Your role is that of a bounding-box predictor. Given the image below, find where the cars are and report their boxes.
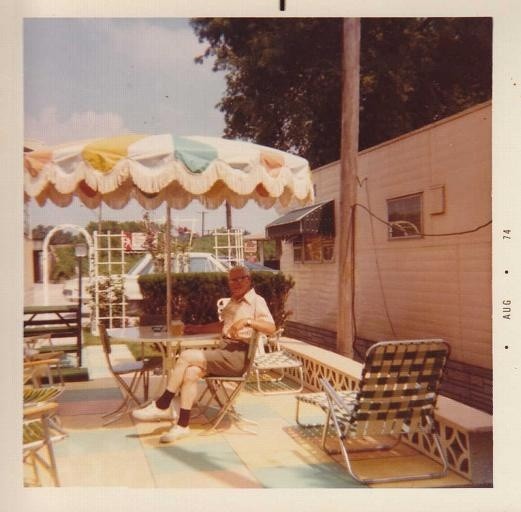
[61,252,280,307]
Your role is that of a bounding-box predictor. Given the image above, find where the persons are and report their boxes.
[130,264,278,443]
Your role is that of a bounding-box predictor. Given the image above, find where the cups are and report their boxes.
[171,318,185,337]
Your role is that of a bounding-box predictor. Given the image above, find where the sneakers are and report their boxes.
[132,400,176,422]
[158,423,193,443]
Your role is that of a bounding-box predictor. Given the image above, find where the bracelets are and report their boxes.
[248,319,252,326]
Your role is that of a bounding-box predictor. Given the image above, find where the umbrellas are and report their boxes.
[24,129,317,387]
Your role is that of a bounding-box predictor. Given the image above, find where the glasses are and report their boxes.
[227,276,248,285]
